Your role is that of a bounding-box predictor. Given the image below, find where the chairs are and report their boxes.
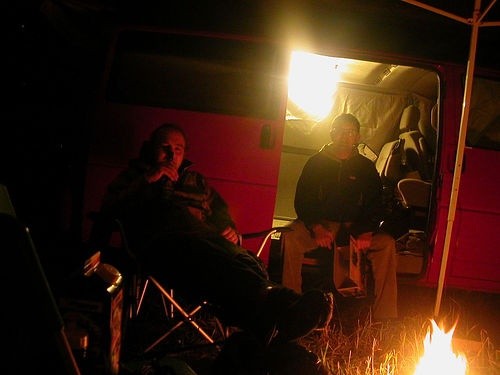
[98,182,243,366]
[373,104,434,226]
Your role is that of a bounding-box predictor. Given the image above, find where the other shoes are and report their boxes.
[272,285,336,346]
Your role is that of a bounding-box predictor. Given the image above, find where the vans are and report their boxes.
[72,26,500,306]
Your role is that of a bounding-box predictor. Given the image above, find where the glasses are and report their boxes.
[334,128,357,142]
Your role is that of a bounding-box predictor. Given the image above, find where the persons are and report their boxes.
[275,114,401,325]
[72,123,337,351]
